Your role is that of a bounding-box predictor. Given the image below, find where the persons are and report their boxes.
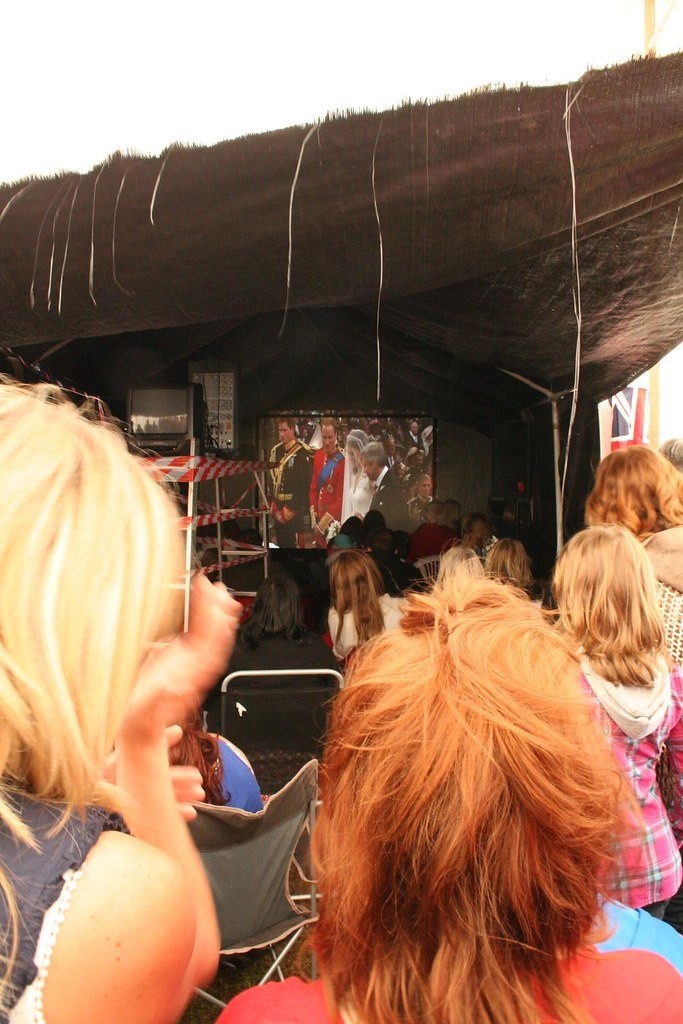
[327,550,410,663]
[355,441,407,531]
[403,474,433,527]
[583,440,683,670]
[486,481,555,595]
[294,417,433,500]
[216,577,683,1024]
[225,574,342,676]
[341,429,374,525]
[309,417,346,549]
[544,522,683,921]
[225,498,534,638]
[165,692,264,813]
[266,418,318,548]
[0,378,222,1024]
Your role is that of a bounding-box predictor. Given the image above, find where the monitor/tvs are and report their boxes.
[128,383,203,440]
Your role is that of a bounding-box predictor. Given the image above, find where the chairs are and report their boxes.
[179,755,321,1010]
[219,669,345,787]
